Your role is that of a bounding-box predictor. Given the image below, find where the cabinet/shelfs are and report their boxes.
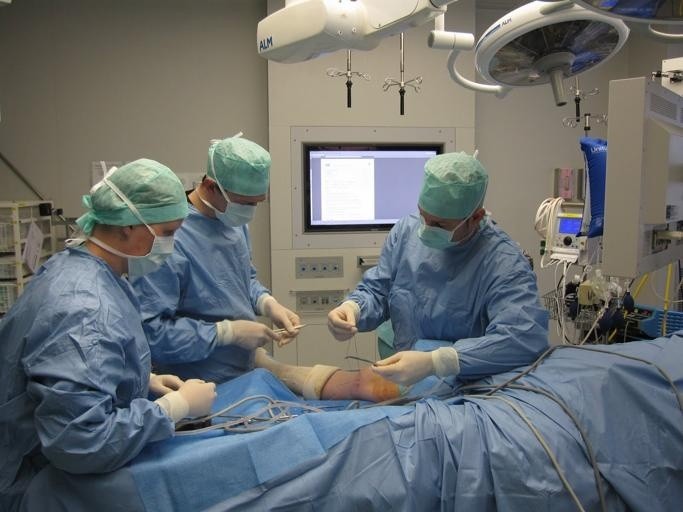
[1,196,56,322]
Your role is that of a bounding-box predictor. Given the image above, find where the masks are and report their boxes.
[416,223,460,250]
[125,235,174,278]
[213,202,257,228]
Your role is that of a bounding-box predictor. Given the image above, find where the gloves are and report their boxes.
[325,300,361,344]
[214,318,281,352]
[369,345,461,388]
[256,292,300,349]
[148,372,184,396]
[154,379,217,424]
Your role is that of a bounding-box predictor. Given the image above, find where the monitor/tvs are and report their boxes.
[304,146,441,231]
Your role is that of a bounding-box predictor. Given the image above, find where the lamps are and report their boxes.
[473,0,633,108]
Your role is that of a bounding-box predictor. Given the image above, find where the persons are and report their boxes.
[0,156,220,512]
[244,343,478,403]
[324,150,551,400]
[114,129,303,402]
